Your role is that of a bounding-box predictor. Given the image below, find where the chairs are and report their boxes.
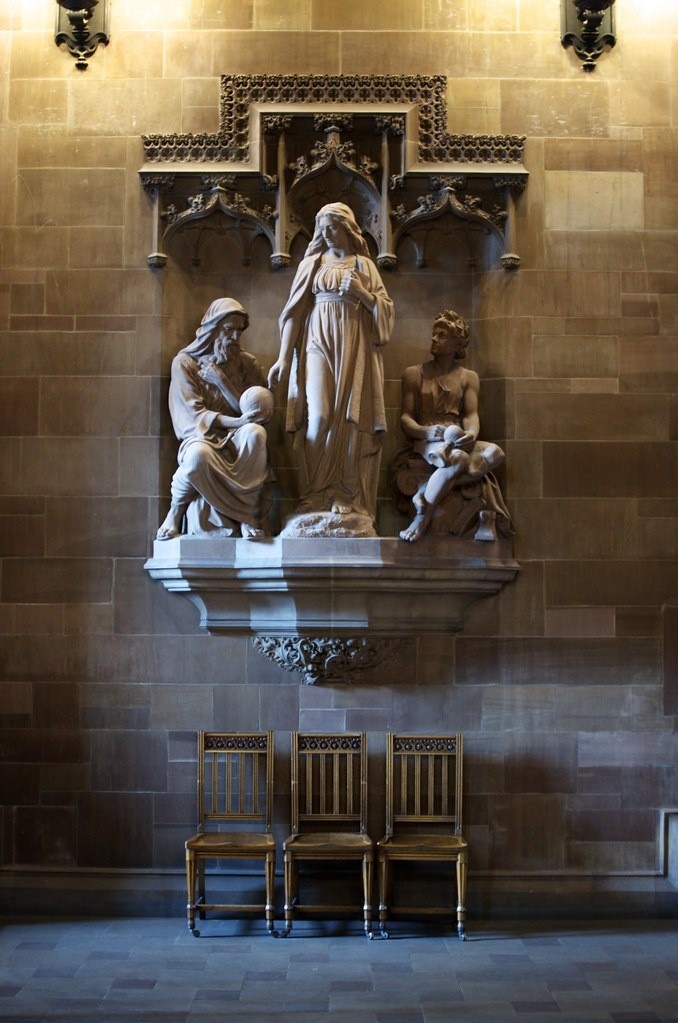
[280,732,375,938]
[376,732,468,942]
[185,730,280,939]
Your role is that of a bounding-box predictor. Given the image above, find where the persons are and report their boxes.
[396,309,507,545]
[156,295,275,542]
[266,200,394,520]
